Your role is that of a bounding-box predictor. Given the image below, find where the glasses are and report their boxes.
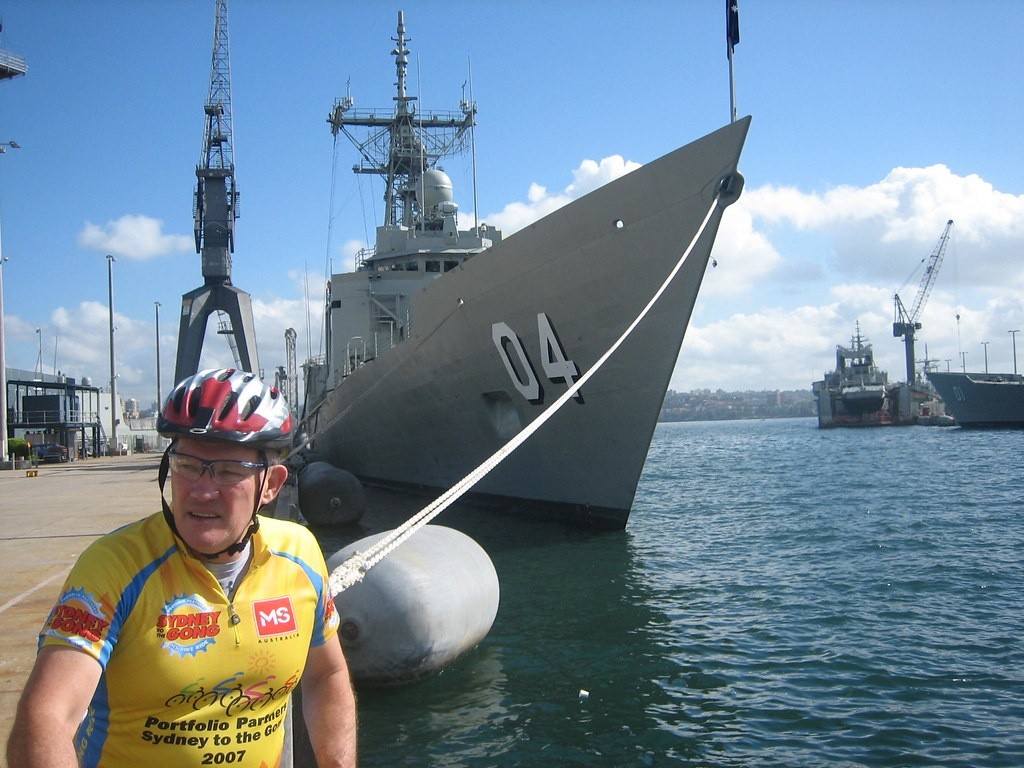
[167,446,266,485]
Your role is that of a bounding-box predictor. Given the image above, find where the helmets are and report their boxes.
[156,369,294,448]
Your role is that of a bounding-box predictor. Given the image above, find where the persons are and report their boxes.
[8,370,360,768]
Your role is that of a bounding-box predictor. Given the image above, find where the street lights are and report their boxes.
[154,302,162,416]
[981,342,990,373]
[106,254,116,453]
[1009,330,1019,374]
[960,352,968,373]
[944,359,952,372]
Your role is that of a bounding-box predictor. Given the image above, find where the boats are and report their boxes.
[926,372,1024,430]
[816,319,895,427]
[289,1,753,535]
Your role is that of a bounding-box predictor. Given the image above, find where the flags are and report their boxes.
[726,0,740,58]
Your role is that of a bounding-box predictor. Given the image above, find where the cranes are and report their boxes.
[894,219,954,387]
